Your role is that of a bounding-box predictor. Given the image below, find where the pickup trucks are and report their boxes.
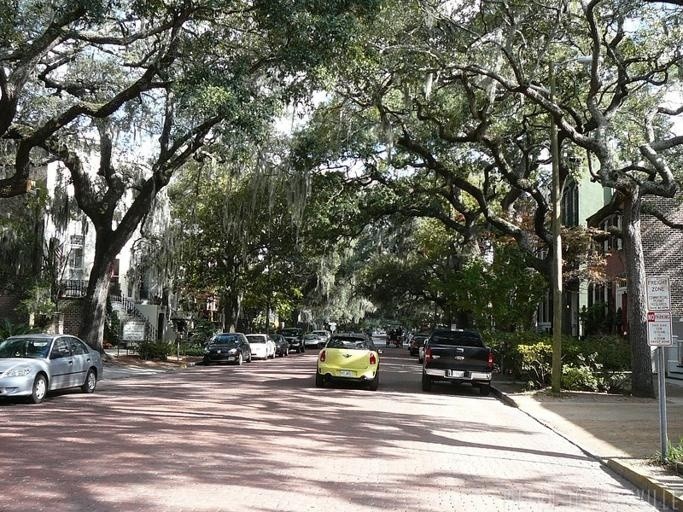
[421,331,496,396]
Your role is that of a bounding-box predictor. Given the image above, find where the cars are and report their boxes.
[408,335,429,363]
[0,334,104,404]
[204,329,332,365]
[315,333,383,391]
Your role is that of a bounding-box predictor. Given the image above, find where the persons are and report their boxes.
[386,325,404,348]
